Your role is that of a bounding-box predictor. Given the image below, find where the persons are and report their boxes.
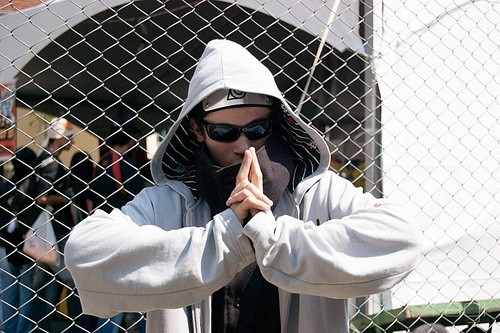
[64,39,423,332]
[0,115,152,331]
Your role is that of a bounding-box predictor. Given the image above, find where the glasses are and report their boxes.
[199,118,275,143]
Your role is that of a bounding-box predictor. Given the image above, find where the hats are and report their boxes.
[192,88,279,117]
[42,116,74,149]
